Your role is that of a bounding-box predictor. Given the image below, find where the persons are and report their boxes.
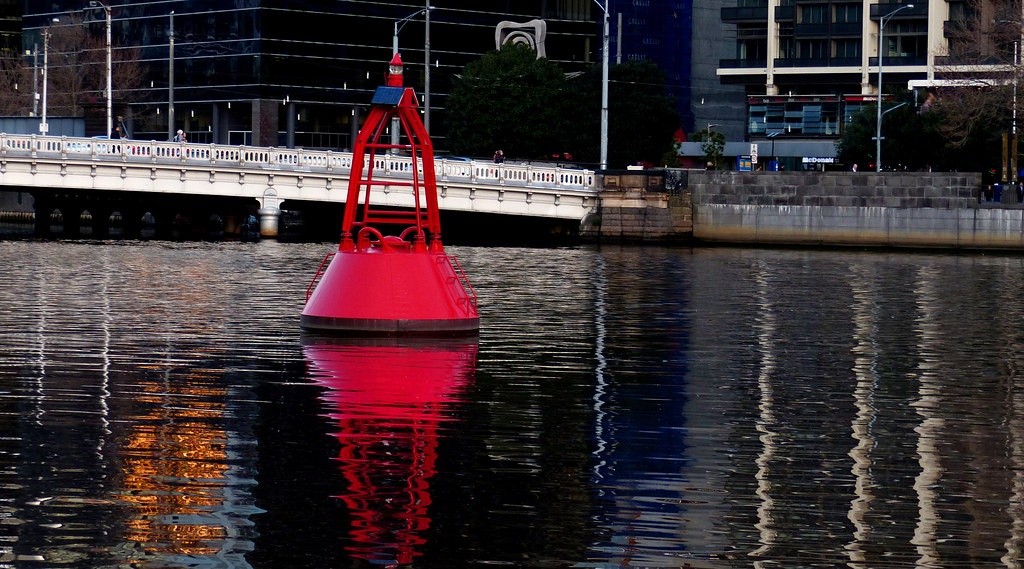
[491,149,504,177]
[110,125,121,153]
[176,129,187,157]
[705,161,715,170]
[850,164,858,172]
[778,164,785,171]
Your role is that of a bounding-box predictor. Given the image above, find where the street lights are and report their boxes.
[392,7,438,153]
[40,19,60,133]
[872,5,914,172]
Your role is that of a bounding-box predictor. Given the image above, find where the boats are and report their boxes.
[300,47,484,334]
[290,330,481,561]
